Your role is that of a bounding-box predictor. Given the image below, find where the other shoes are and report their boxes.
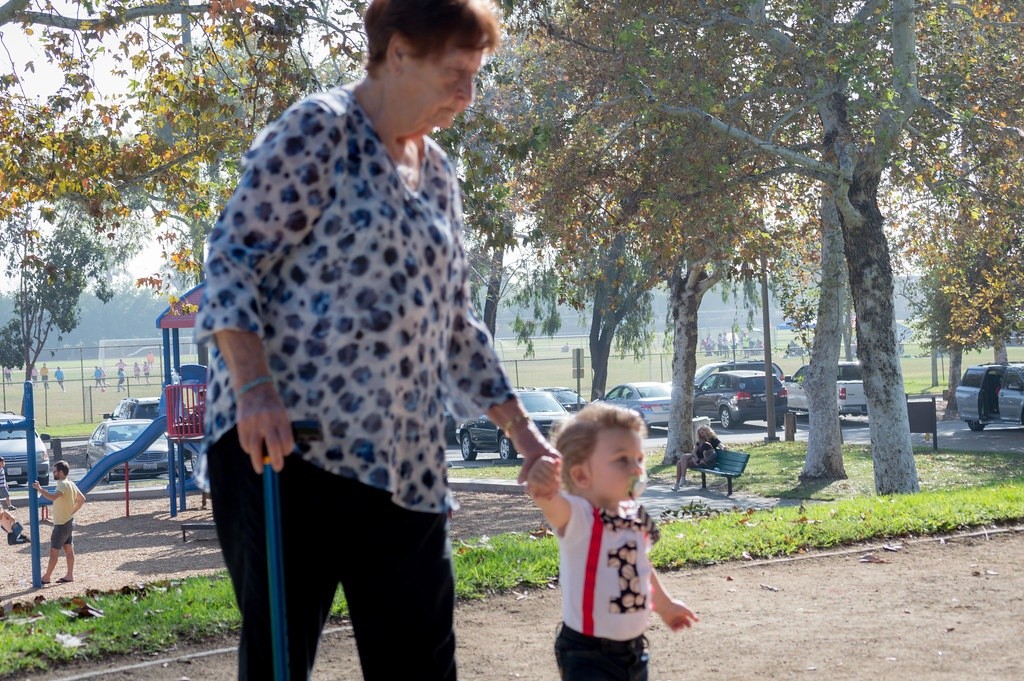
[671,484,680,491]
[679,478,687,487]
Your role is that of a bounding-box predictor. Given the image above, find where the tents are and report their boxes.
[774,317,818,356]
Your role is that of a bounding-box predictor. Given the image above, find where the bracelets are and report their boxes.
[234,378,274,399]
[503,415,532,437]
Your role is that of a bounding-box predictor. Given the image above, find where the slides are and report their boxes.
[37,413,168,506]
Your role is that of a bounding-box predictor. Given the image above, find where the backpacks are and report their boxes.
[697,442,718,469]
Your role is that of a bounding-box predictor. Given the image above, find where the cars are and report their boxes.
[86,416,180,485]
[181,408,204,472]
[542,386,587,413]
[594,382,671,429]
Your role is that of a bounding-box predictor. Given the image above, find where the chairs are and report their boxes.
[108,431,118,442]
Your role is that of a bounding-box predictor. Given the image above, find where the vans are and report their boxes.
[0,415,51,486]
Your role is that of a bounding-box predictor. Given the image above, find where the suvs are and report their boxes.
[694,359,783,388]
[694,371,789,430]
[459,387,570,462]
[128,347,164,357]
[103,396,189,422]
[781,362,868,417]
[954,361,1024,431]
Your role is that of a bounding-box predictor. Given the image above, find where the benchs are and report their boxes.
[687,448,751,496]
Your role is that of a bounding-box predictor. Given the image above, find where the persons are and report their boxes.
[672,426,730,491]
[0,458,86,584]
[200,0,566,681]
[701,333,813,357]
[5,351,154,392]
[525,404,699,681]
[992,378,1002,412]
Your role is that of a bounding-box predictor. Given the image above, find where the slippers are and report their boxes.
[56,578,72,583]
[41,581,50,584]
